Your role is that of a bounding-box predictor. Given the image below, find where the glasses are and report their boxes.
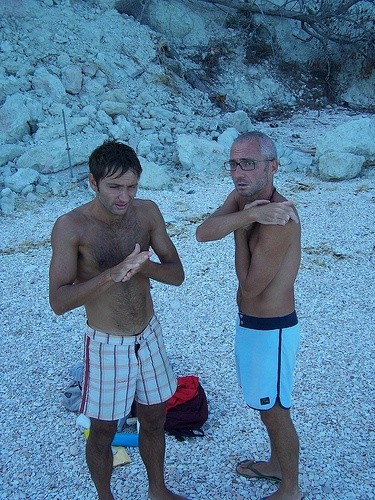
[224,160,272,171]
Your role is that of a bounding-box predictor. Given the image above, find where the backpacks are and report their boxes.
[164,376,209,442]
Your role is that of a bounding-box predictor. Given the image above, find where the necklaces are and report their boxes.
[268,187,277,204]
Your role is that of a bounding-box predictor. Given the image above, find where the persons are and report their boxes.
[193,131,305,500]
[46,140,192,500]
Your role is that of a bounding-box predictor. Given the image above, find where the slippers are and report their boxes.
[236,459,282,483]
